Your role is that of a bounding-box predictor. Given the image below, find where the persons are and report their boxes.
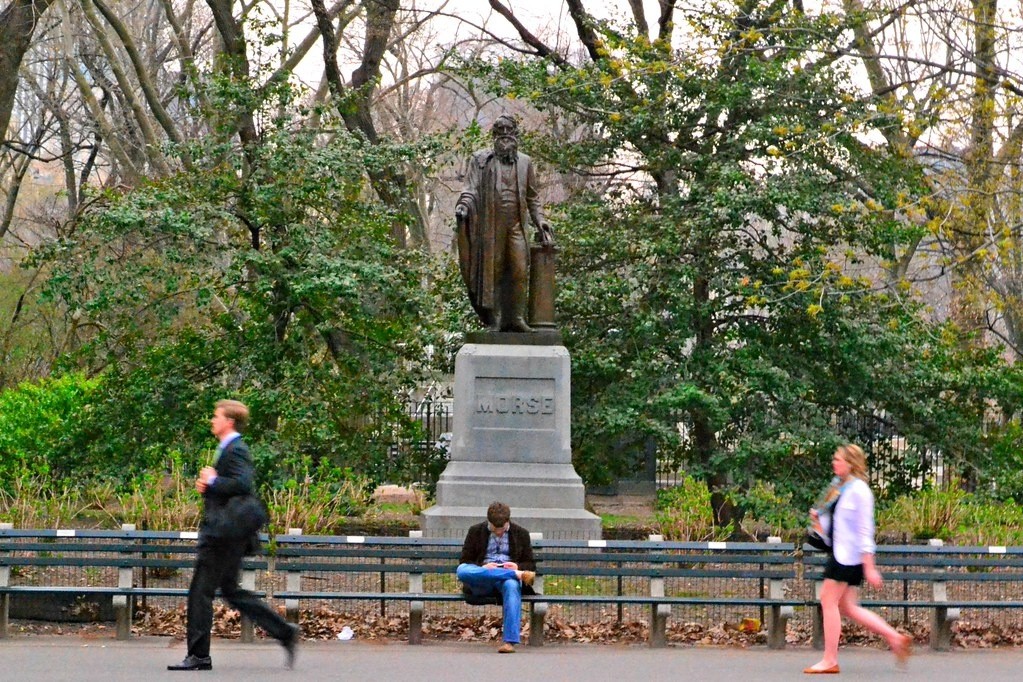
[165,399,300,672]
[453,114,555,332]
[803,444,910,674]
[455,502,536,653]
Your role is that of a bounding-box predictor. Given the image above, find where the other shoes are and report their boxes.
[803,664,840,674]
[896,634,914,665]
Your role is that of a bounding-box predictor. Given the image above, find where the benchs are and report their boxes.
[0,522,274,643]
[799,539,1023,649]
[274,528,807,649]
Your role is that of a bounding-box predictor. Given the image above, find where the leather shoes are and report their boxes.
[521,570,536,586]
[281,622,299,668]
[166,655,214,671]
[496,642,516,653]
